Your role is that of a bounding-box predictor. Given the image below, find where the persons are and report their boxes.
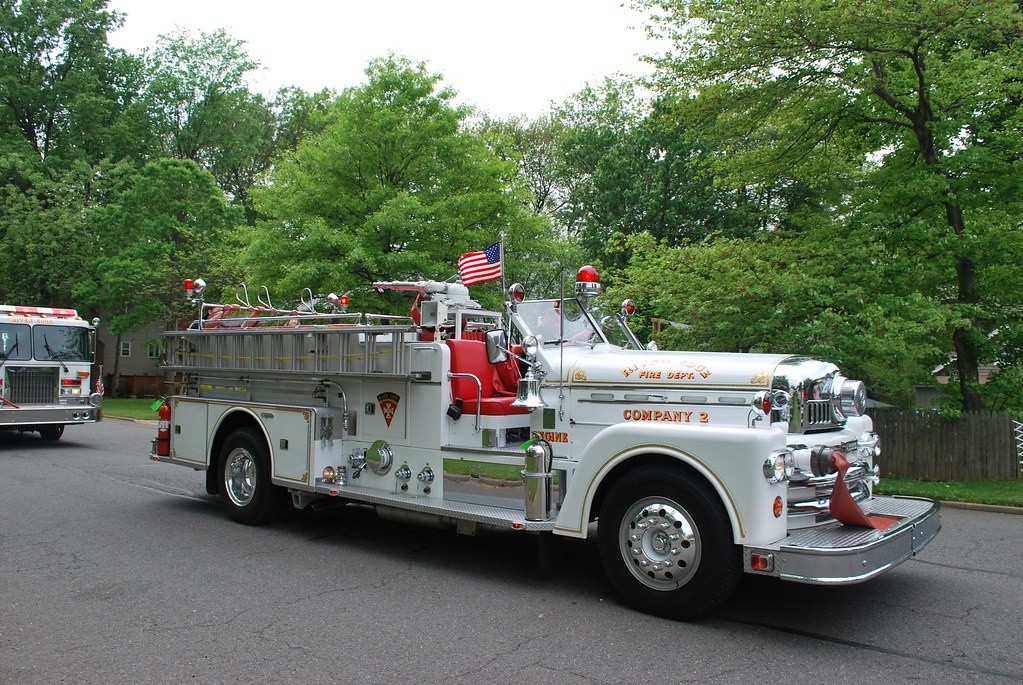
[536,300,592,342]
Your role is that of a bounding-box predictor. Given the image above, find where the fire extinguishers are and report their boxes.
[156,394,172,455]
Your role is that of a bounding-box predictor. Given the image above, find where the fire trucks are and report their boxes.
[0,304,105,439]
[150,266,946,623]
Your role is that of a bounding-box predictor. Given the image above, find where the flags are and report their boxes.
[457,242,503,285]
[96,376,104,397]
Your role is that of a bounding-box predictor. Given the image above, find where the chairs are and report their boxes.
[444,339,530,415]
[496,345,529,394]
